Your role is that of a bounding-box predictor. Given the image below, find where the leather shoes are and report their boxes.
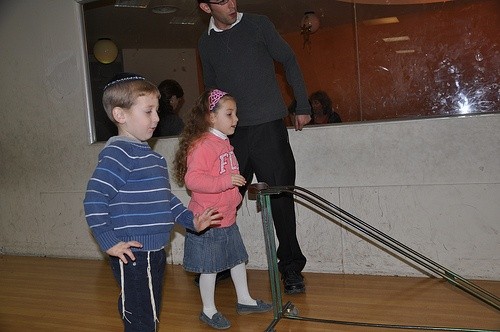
[283,274,304,294]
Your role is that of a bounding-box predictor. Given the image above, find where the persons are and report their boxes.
[288,90,343,126]
[153,79,185,137]
[83,73,224,332]
[171,88,274,331]
[193,0,313,296]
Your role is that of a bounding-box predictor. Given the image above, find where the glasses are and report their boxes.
[203,0,230,5]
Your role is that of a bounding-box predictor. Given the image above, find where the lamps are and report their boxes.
[93,37,119,63]
[301,12,320,33]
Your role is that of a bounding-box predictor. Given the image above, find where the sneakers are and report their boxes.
[199,309,232,330]
[236,298,272,316]
